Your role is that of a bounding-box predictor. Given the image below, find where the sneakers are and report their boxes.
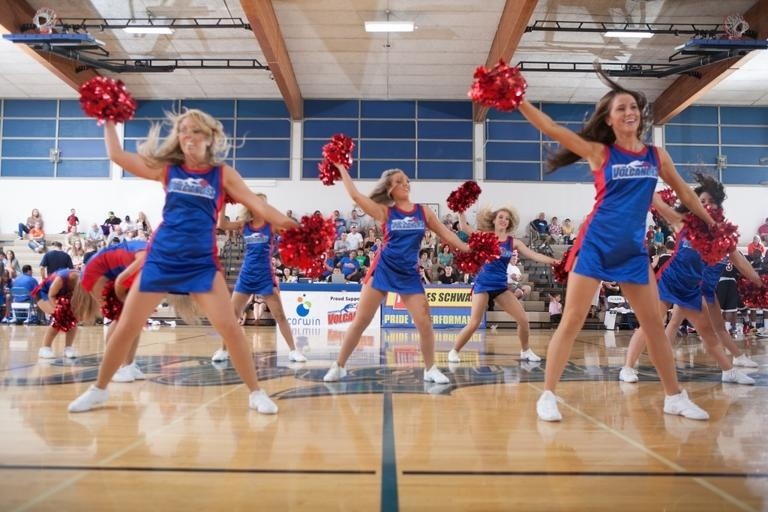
[521,348,541,361]
[323,363,346,382]
[448,351,459,363]
[111,364,146,383]
[664,390,709,420]
[68,390,108,411]
[424,365,449,383]
[537,391,562,421]
[289,349,307,362]
[722,354,758,384]
[212,350,227,361]
[619,367,638,383]
[249,391,277,413]
[63,348,75,357]
[38,347,55,359]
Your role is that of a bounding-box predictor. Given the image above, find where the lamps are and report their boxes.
[362,9,418,34]
[602,17,655,39]
[121,10,176,37]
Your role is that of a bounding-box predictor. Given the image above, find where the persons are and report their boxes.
[211,193,308,362]
[31,269,100,359]
[67,106,299,413]
[518,58,718,422]
[0,208,768,338]
[81,240,200,383]
[619,171,764,384]
[665,255,759,368]
[447,204,561,363]
[322,161,473,385]
